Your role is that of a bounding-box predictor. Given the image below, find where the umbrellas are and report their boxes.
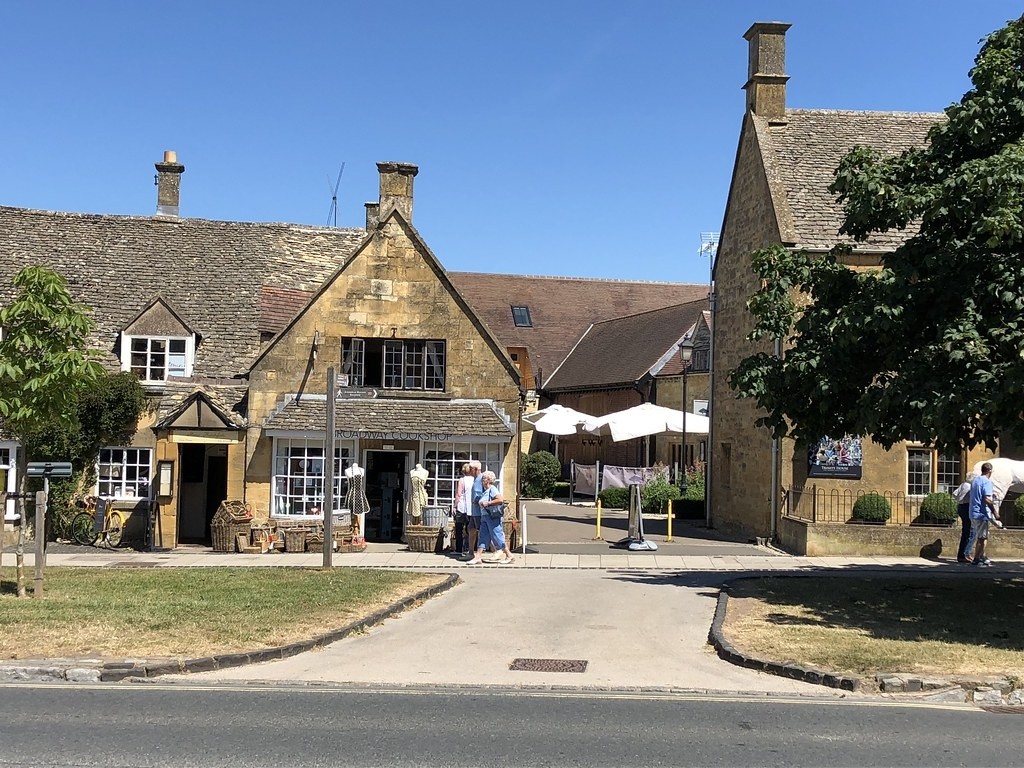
[521,403,595,456]
[581,402,709,466]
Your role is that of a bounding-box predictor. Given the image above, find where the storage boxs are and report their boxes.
[236,532,262,554]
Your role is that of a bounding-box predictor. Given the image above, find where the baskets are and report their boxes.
[404,520,445,552]
[210,500,367,554]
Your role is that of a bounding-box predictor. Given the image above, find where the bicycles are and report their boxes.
[71,494,127,548]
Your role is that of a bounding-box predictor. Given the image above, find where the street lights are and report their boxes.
[677,332,694,499]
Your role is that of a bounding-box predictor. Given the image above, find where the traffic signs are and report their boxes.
[334,387,378,400]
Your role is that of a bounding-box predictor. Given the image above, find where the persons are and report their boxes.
[452,459,516,564]
[969,463,1001,566]
[344,463,371,515]
[952,471,979,563]
[816,434,855,466]
[405,464,429,516]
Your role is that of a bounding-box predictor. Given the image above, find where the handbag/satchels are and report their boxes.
[484,489,505,518]
[455,510,469,523]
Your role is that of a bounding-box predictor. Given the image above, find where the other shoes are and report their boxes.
[500,554,516,564]
[971,556,995,567]
[956,555,974,563]
[450,550,464,555]
[459,554,475,560]
[466,558,482,565]
